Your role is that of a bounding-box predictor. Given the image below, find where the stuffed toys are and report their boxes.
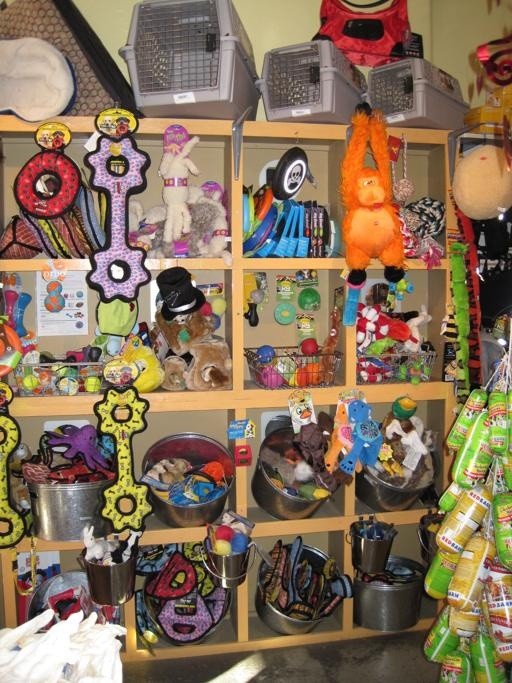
[338,109,408,289]
[324,393,384,475]
[356,272,436,386]
[150,266,231,390]
[129,123,233,268]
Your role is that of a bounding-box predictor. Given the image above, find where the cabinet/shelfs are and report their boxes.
[0,114,512,666]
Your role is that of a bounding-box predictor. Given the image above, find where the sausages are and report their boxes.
[422,387,512,683]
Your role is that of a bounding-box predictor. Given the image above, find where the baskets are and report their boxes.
[244,346,343,389]
[357,352,438,383]
[13,360,105,397]
